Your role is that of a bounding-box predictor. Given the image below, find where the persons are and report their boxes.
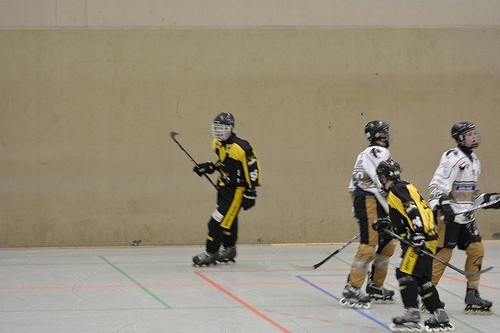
[191,111,262,267]
[420,121,500,316]
[372,157,455,332]
[339,120,398,309]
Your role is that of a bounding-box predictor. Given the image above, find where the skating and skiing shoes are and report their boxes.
[339,274,371,309]
[390,306,423,332]
[419,297,445,314]
[464,288,493,316]
[216,245,237,263]
[366,271,395,304]
[423,308,454,332]
[192,250,218,267]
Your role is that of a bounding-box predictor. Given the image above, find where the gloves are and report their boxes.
[481,193,500,209]
[372,216,393,231]
[410,232,426,256]
[241,191,257,210]
[440,195,455,224]
[193,161,215,177]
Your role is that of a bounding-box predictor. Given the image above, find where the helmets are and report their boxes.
[451,121,475,136]
[211,112,235,141]
[376,158,400,179]
[365,120,388,141]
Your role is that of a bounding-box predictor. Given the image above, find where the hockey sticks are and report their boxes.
[439,197,500,222]
[288,232,361,272]
[169,130,220,191]
[381,228,493,276]
